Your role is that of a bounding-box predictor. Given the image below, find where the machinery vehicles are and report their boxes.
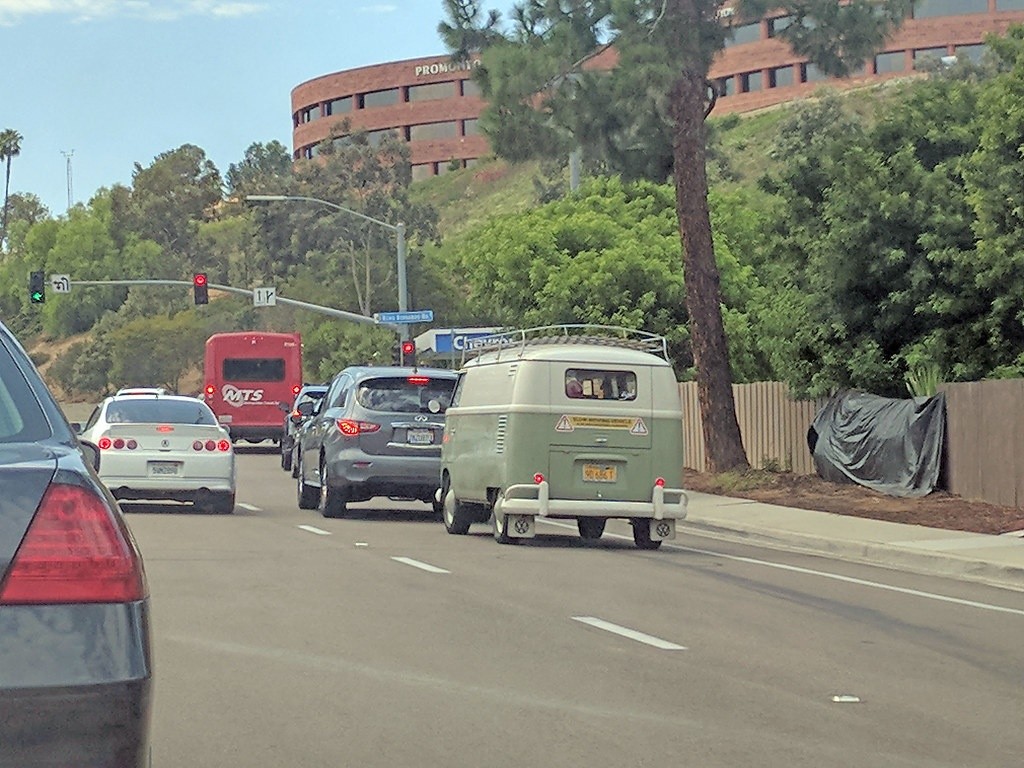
[60,147,77,210]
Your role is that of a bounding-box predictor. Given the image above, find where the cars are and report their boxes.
[69,393,236,514]
[1,321,158,768]
[438,323,692,552]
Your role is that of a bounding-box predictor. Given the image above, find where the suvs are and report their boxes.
[116,387,171,396]
[294,365,459,519]
[279,381,329,470]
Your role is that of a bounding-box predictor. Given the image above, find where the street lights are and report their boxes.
[244,192,415,365]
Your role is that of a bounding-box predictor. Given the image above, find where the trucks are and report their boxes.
[203,330,303,450]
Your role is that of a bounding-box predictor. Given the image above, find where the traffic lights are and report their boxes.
[30,271,47,305]
[194,272,209,305]
[403,341,415,366]
